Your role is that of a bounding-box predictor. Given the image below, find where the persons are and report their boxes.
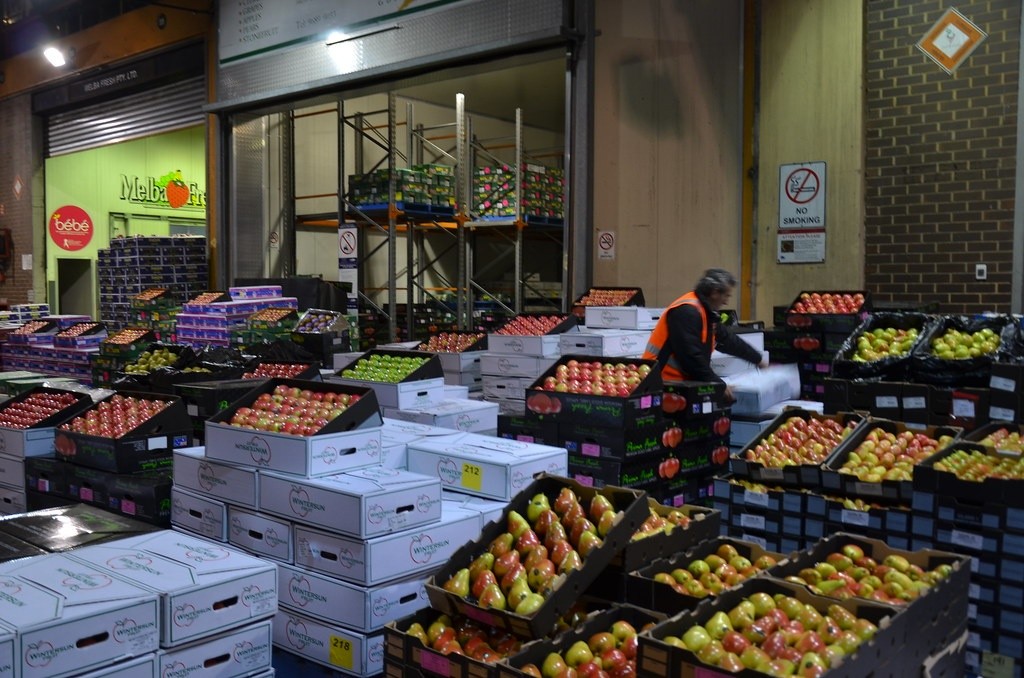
[644,269,767,407]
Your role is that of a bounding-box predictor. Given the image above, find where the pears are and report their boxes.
[400,484,960,678]
[730,325,1024,510]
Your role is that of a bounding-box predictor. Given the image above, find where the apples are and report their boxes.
[789,292,867,314]
[0,289,652,440]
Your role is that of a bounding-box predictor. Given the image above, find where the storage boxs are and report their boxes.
[0,163,1024,678]
